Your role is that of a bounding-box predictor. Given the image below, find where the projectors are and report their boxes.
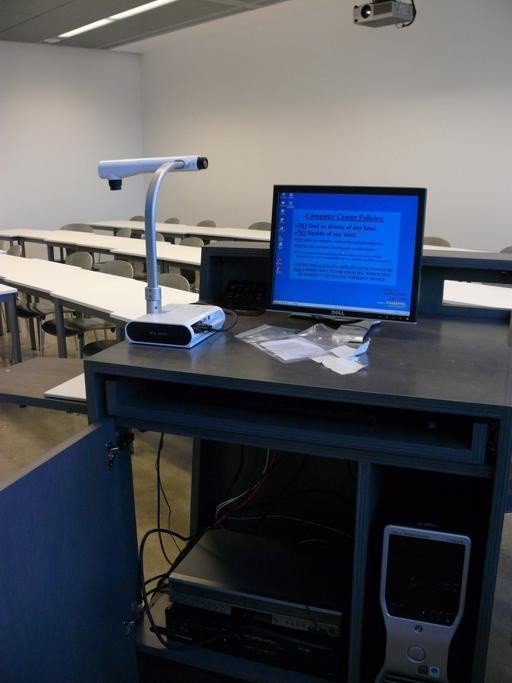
[353,0,415,28]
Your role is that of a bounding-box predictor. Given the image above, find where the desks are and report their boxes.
[79,236,512,683]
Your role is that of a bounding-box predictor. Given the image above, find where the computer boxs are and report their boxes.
[359,500,489,683]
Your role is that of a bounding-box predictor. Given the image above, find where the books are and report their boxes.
[43,372,87,402]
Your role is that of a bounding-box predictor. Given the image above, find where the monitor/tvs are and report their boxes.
[267,184,427,325]
